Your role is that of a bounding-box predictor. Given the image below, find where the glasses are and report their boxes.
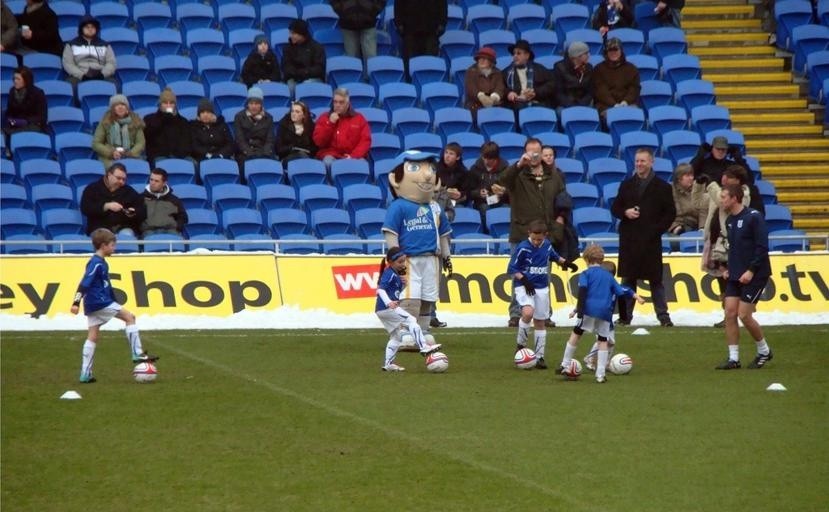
[512,52,523,55]
[608,47,620,51]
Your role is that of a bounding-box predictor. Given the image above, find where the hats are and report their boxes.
[675,163,692,177]
[722,165,747,179]
[712,136,728,149]
[474,39,622,58]
[255,35,271,47]
[108,86,265,115]
[288,19,309,37]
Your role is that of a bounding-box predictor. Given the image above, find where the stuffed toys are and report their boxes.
[381,150,452,347]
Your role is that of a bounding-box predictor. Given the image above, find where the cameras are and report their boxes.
[532,152,542,160]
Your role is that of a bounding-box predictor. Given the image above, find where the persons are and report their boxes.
[88,89,371,160]
[70,227,160,384]
[394,1,447,81]
[80,164,146,235]
[138,168,186,234]
[376,247,442,371]
[611,147,677,326]
[437,141,471,207]
[691,164,773,370]
[669,136,756,235]
[282,20,323,83]
[557,245,646,384]
[0,0,119,109]
[499,138,567,328]
[506,220,579,369]
[470,142,508,235]
[464,40,640,108]
[653,0,685,25]
[243,34,280,88]
[1,66,58,161]
[595,0,631,31]
[330,0,386,81]
[431,189,455,328]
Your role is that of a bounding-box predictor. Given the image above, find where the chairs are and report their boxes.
[761,0,829,132]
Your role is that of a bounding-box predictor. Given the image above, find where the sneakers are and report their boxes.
[555,365,564,374]
[79,374,95,382]
[661,321,674,327]
[614,319,630,325]
[605,362,610,371]
[594,376,607,383]
[747,350,772,369]
[715,358,742,370]
[544,318,555,327]
[382,364,406,372]
[514,344,524,354]
[535,357,548,369]
[509,318,519,327]
[714,320,727,328]
[420,344,442,357]
[399,342,420,352]
[430,319,447,328]
[132,352,159,363]
[584,356,596,370]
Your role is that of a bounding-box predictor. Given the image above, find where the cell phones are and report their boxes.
[634,205,640,211]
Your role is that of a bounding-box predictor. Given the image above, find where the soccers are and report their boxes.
[515,348,537,370]
[426,352,448,372]
[610,353,632,374]
[134,363,157,382]
[566,359,582,378]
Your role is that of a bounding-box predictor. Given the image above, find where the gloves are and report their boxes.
[673,225,683,235]
[520,276,535,295]
[703,174,711,186]
[562,260,578,273]
[696,175,704,184]
